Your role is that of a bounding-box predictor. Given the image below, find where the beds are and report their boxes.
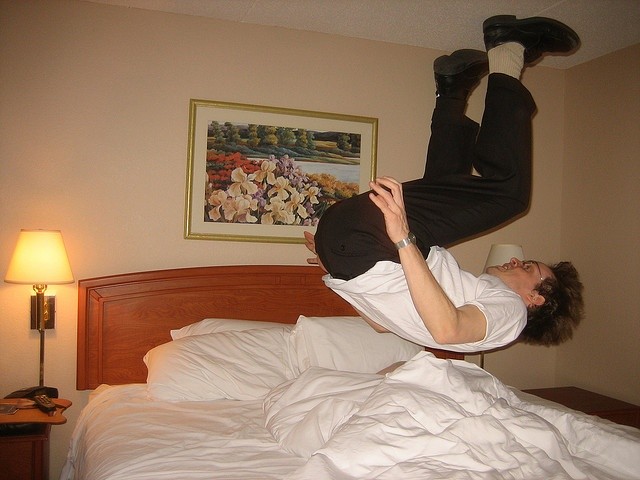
[76,265,639,480]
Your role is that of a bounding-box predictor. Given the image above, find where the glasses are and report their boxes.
[521,260,543,282]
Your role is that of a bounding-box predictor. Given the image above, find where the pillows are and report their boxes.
[170,318,294,343]
[289,314,424,380]
[142,326,291,402]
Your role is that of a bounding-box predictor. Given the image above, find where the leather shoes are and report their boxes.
[483,15,580,65]
[433,48,488,94]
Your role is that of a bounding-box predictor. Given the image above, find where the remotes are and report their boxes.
[35,394,56,412]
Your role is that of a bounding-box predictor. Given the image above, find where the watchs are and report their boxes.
[394,232,418,248]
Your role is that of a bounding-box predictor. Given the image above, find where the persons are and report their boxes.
[305,15,586,354]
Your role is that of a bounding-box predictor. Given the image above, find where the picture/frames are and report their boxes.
[183,98,378,244]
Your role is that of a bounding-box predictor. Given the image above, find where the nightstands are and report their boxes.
[520,386,640,429]
[0,398,72,480]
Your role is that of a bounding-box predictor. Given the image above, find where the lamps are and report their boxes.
[3,229,75,396]
[478,243,525,369]
